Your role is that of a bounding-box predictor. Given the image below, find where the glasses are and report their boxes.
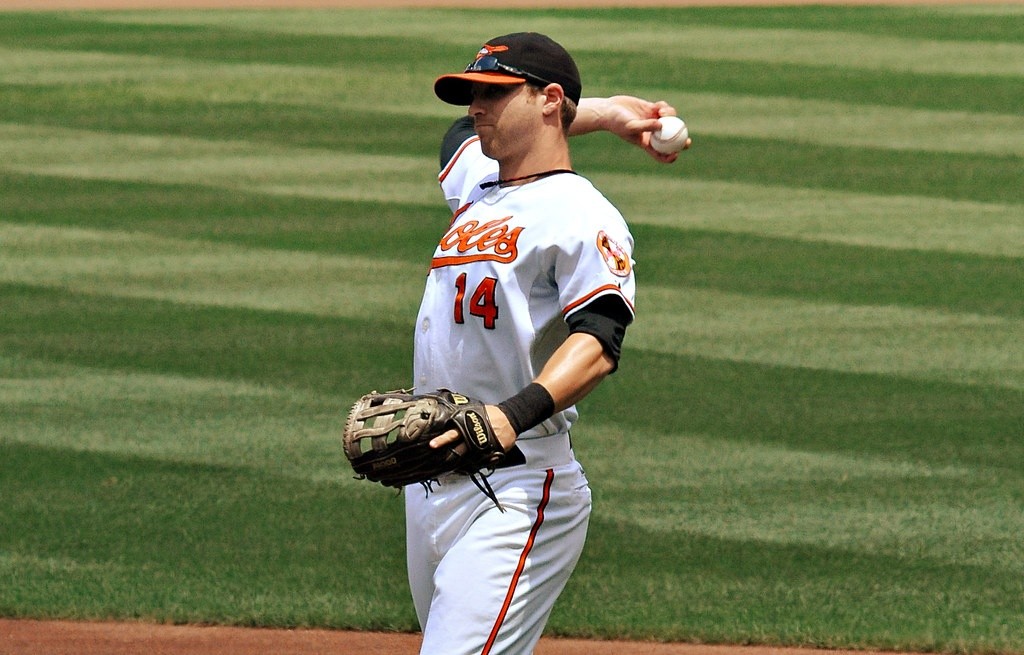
[464,56,550,84]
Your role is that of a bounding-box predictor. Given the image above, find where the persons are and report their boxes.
[342,33,692,655]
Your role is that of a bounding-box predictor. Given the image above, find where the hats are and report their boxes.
[433,32,583,106]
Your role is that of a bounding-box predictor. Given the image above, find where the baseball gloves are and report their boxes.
[341,386,528,516]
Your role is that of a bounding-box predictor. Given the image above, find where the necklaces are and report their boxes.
[479,170,577,193]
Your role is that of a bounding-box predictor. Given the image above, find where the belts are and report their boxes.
[455,431,572,473]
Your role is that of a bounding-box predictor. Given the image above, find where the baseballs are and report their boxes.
[648,115,688,155]
